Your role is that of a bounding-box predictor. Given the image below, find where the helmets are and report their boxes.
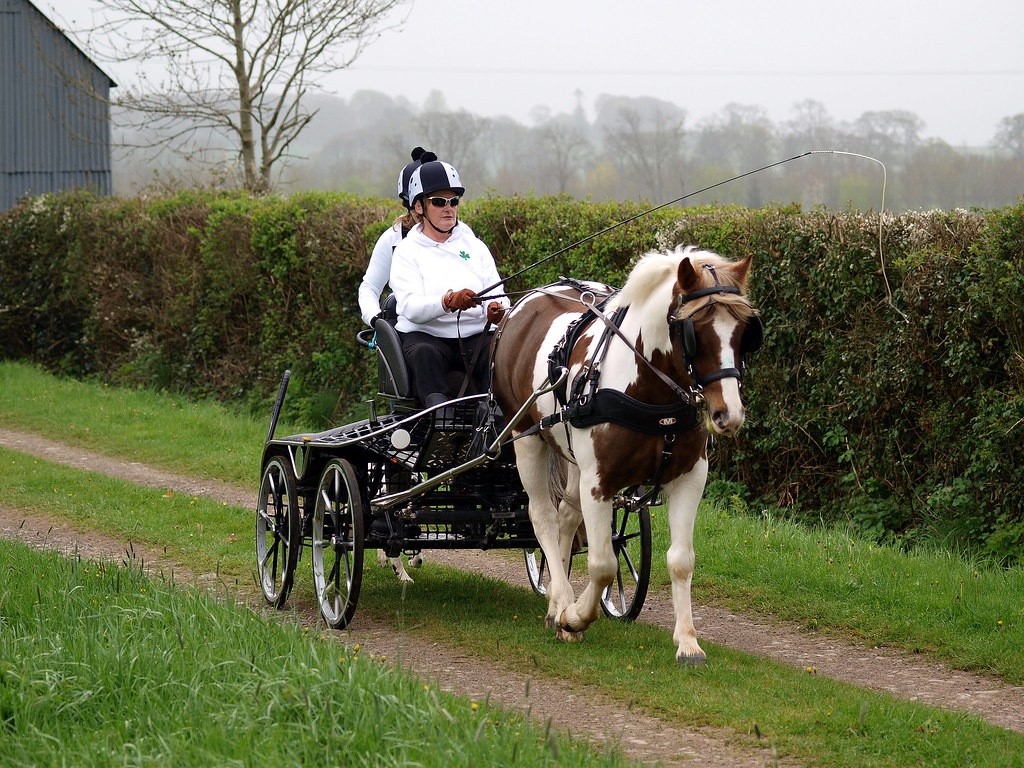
[408,151,466,234]
[397,147,426,210]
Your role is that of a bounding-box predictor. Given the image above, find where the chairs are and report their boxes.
[356,296,484,415]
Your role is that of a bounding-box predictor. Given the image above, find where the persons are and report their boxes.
[356,146,476,539]
[387,152,512,451]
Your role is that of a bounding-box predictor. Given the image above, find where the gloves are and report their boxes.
[371,313,397,328]
[487,301,505,324]
[444,288,479,313]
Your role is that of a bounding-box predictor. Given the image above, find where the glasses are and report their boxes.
[427,196,460,207]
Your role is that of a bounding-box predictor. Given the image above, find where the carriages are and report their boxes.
[257,242,765,662]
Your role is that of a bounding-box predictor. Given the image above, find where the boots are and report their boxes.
[426,394,468,444]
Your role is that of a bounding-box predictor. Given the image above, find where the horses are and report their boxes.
[490,241,760,665]
